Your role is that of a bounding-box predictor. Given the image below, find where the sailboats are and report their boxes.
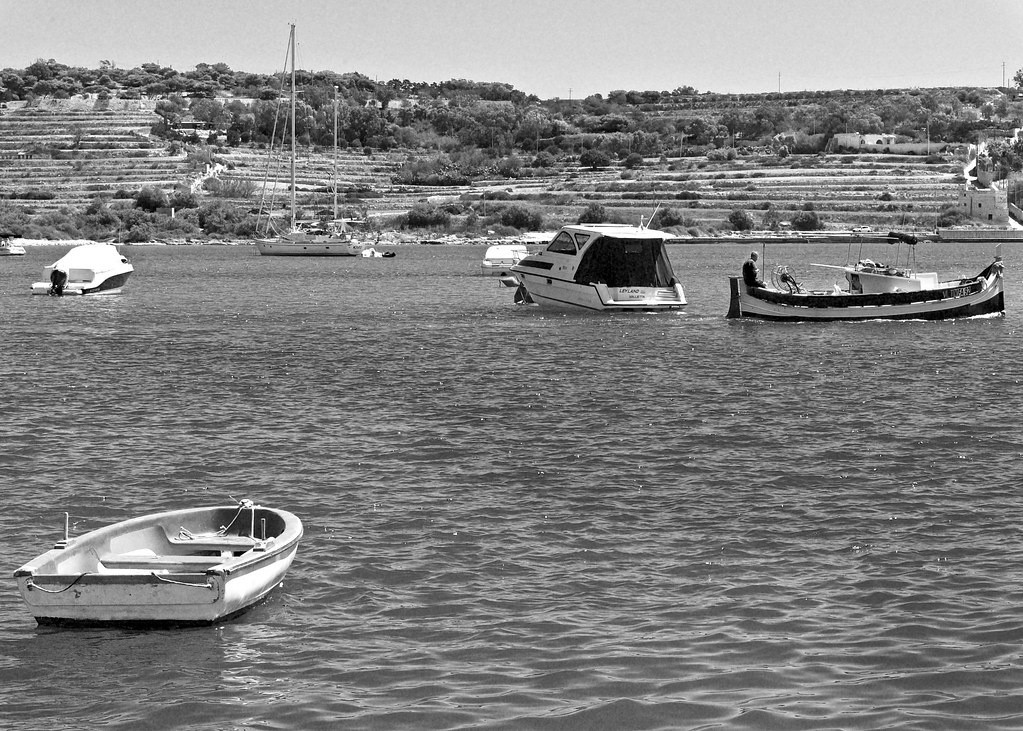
[251,23,365,258]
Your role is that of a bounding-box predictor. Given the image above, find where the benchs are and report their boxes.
[98,555,238,572]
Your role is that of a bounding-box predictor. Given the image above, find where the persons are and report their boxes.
[323,228,328,235]
[742,251,768,289]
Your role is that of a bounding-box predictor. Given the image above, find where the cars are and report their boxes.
[852,225,871,233]
[0,237,27,257]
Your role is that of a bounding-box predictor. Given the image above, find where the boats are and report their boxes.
[108,236,259,246]
[508,203,687,312]
[12,493,306,625]
[726,245,1008,327]
[371,226,557,245]
[478,242,532,287]
[29,241,134,296]
